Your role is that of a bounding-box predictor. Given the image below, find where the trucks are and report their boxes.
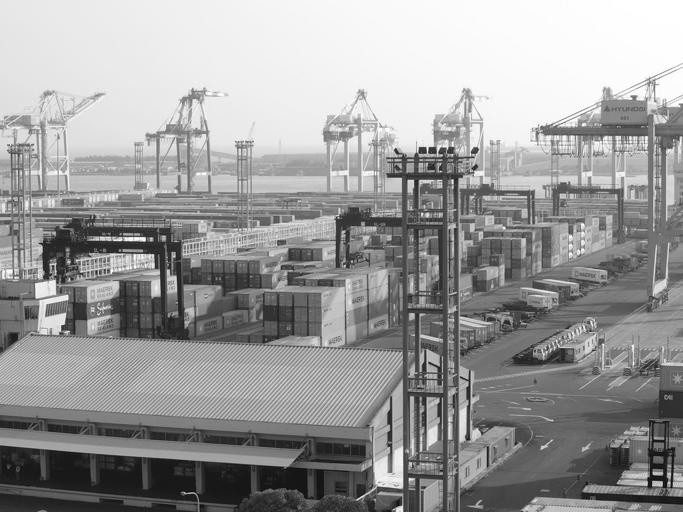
[512,315,597,364]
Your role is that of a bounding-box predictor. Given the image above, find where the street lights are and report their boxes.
[180,491,201,512]
